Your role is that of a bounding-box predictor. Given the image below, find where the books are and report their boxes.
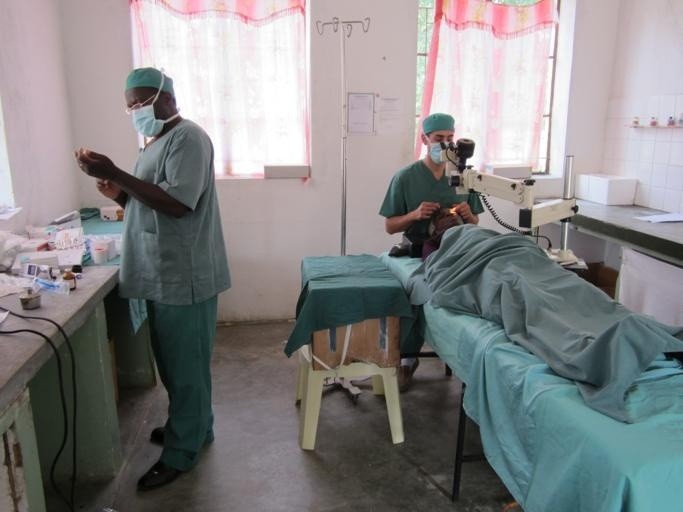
[11,249,82,274]
[548,248,590,271]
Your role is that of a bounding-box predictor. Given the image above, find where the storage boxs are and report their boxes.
[576,170,638,207]
[303,254,401,369]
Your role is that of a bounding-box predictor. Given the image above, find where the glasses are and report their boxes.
[124,95,155,114]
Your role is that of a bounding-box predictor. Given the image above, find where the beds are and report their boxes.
[381,245,681,509]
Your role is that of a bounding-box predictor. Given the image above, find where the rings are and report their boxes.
[79,164,82,167]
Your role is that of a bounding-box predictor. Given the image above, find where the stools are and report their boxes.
[298,342,403,453]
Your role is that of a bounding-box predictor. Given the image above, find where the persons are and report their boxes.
[73,67,231,492]
[377,113,485,392]
[423,208,464,259]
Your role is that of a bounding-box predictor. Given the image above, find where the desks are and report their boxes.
[1,265,120,511]
[75,210,158,393]
[530,193,681,329]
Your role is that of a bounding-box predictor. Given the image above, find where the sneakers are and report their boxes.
[137,458,188,491]
[151,427,215,447]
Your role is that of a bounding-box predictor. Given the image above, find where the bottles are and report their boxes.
[62,267,77,291]
[19,285,41,310]
[631,114,683,126]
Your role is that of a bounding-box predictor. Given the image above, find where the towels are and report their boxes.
[544,247,587,272]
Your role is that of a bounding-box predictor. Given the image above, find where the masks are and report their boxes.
[429,142,453,165]
[131,105,164,138]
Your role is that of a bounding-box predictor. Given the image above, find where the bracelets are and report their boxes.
[113,190,129,208]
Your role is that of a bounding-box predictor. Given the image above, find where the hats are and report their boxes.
[125,67,175,97]
[422,113,455,136]
